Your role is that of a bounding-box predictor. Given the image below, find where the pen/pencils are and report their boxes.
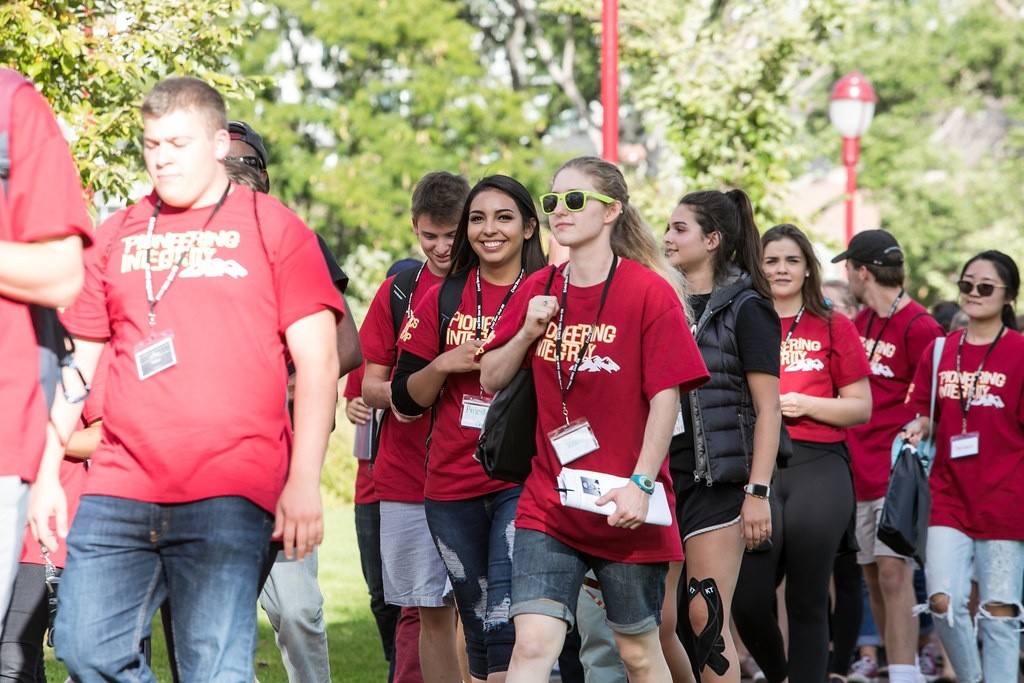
[554,487,575,491]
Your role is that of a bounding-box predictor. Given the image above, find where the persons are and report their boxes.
[0,65,97,627]
[0,80,361,683]
[343,156,1024,683]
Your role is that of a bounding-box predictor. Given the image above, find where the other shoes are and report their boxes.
[846,656,879,682]
[919,646,945,681]
[827,666,843,681]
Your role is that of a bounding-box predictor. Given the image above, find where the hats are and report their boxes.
[227,119,271,195]
[385,258,423,278]
[831,229,904,267]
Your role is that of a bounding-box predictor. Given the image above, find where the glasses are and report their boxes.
[540,189,623,216]
[230,156,263,175]
[958,280,1012,297]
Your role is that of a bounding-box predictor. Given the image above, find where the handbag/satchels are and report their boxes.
[472,345,535,485]
[872,436,929,572]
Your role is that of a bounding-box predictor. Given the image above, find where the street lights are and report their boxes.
[827,70,877,247]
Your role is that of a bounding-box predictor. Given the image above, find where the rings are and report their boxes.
[544,301,548,306]
[760,528,767,531]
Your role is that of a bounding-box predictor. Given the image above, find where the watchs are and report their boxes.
[743,483,770,499]
[631,474,655,494]
